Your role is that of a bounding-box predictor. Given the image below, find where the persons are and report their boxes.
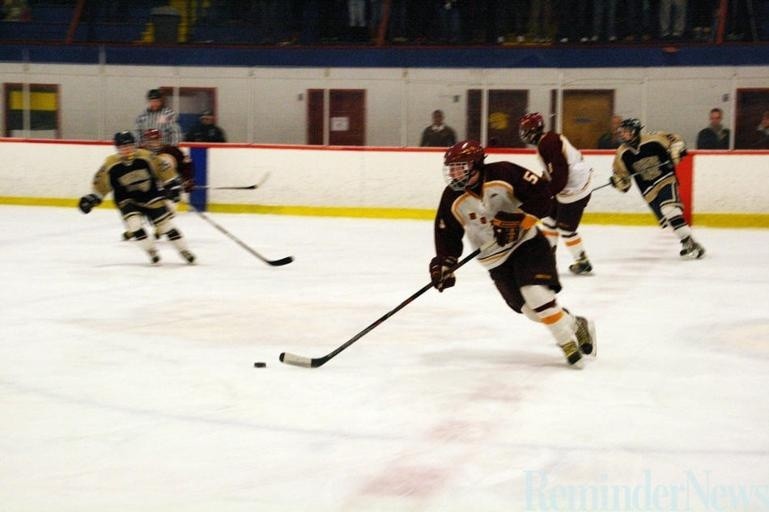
[748,112,769,149]
[182,108,226,142]
[608,118,706,257]
[517,112,592,276]
[130,90,179,154]
[596,116,623,149]
[121,129,195,241]
[697,109,729,149]
[78,133,196,264]
[418,110,455,147]
[430,140,598,369]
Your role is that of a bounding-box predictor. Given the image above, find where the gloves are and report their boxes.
[492,210,525,247]
[78,194,103,212]
[168,179,196,202]
[430,255,456,291]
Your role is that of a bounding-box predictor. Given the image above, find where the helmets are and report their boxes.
[442,140,484,191]
[113,89,217,157]
[520,111,544,143]
[617,117,643,146]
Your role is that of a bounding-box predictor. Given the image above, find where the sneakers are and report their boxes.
[122,229,195,263]
[557,237,704,365]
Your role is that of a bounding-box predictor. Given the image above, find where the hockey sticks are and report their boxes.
[178,198,295,266]
[192,173,268,190]
[279,235,499,368]
